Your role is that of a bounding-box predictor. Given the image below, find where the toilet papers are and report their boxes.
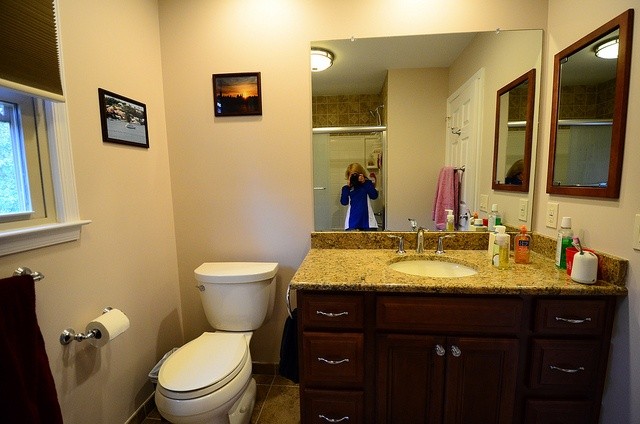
[85,308,130,347]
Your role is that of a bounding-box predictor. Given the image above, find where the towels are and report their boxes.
[432,166,459,230]
[0,275,63,424]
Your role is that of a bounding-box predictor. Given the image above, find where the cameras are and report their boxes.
[350,173,360,187]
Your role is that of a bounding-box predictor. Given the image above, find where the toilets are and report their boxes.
[155,262,278,424]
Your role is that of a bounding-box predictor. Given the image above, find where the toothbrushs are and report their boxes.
[572,237,584,255]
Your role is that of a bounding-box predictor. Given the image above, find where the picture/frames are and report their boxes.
[211,72,263,118]
[98,88,150,149]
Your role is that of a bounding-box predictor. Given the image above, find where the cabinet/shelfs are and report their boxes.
[298,291,369,423]
[523,297,609,423]
[374,292,523,424]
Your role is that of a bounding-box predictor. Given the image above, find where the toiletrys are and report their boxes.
[571,242,579,252]
[471,212,478,225]
[555,216,573,269]
[493,225,510,269]
[488,204,502,232]
[469,219,486,231]
[514,225,532,263]
[445,210,454,232]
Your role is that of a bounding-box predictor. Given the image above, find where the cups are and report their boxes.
[566,247,594,276]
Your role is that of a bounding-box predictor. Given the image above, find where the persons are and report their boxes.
[340,162,379,231]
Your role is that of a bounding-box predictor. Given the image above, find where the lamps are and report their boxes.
[311,46,336,72]
[595,38,619,60]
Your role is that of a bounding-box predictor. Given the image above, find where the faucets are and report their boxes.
[408,218,417,232]
[416,228,430,253]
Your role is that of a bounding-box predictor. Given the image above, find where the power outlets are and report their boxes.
[545,200,559,229]
[518,199,528,221]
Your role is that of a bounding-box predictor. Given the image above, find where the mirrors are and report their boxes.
[310,29,542,233]
[492,68,536,192]
[545,7,635,200]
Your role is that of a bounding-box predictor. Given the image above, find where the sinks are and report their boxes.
[387,255,479,278]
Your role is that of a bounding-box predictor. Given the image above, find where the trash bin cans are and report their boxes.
[148,347,179,390]
